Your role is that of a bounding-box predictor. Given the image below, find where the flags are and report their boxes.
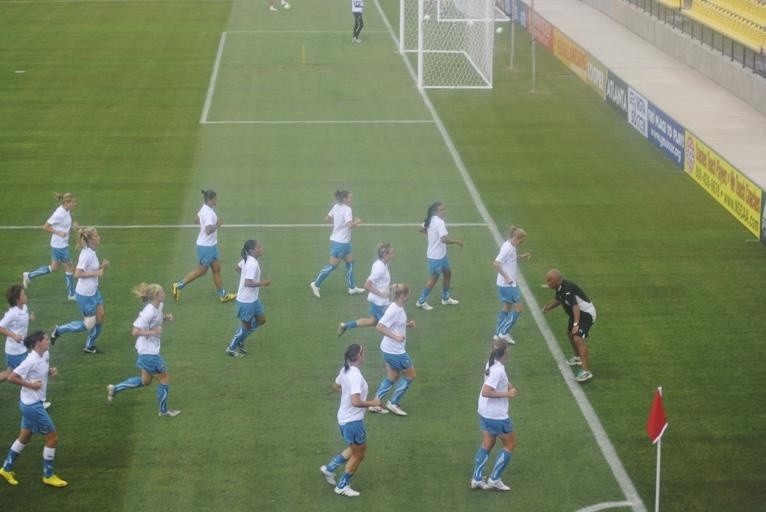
[642,386,670,447]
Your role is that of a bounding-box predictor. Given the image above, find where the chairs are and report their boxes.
[625,0,766,78]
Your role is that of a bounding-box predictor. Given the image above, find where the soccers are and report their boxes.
[285,3,290,9]
[496,27,504,34]
[467,20,473,26]
[423,14,431,21]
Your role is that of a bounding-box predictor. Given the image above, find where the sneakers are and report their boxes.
[308,281,366,298]
[368,399,409,417]
[471,477,512,492]
[224,340,249,359]
[0,465,68,488]
[170,282,237,303]
[336,321,348,337]
[104,382,183,418]
[49,324,98,354]
[565,354,594,382]
[318,465,361,499]
[492,332,517,346]
[415,297,460,310]
[21,270,30,289]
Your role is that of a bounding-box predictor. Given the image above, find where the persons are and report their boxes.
[266,0,290,13]
[48,224,112,356]
[333,240,398,339]
[0,284,35,384]
[469,335,517,492]
[308,188,367,300]
[416,201,466,311]
[317,342,383,496]
[540,268,598,383]
[20,191,77,300]
[350,0,366,45]
[491,223,533,346]
[366,283,418,417]
[226,239,274,360]
[106,281,182,418]
[0,330,69,488]
[171,188,238,303]
